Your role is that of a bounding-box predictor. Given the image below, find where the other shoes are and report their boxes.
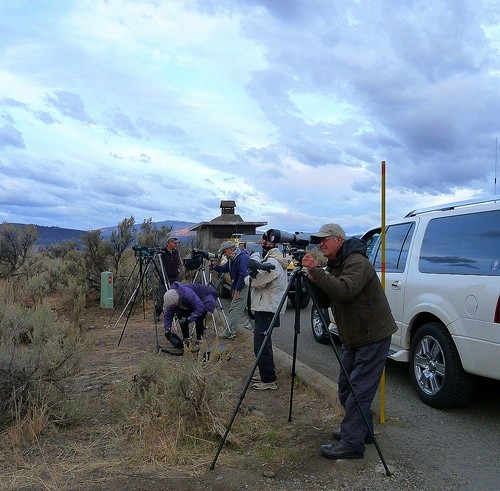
[219,331,236,338]
[191,341,203,353]
[244,324,252,330]
[181,338,190,347]
[155,314,160,321]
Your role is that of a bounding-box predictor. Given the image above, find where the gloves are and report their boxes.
[178,317,189,326]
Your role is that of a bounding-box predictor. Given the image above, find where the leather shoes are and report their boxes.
[333,430,373,444]
[320,441,364,459]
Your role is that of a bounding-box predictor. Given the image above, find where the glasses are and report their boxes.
[318,238,335,244]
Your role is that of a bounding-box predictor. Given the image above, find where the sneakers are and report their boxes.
[251,376,261,382]
[252,381,277,390]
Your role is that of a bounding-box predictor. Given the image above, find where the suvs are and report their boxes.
[311,196,499,409]
[219,241,310,310]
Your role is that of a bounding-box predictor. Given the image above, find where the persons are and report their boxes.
[153,238,183,322]
[209,241,262,340]
[303,223,398,461]
[162,281,217,351]
[245,234,291,390]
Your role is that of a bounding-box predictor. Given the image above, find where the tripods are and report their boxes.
[107,252,182,346]
[192,267,233,340]
[209,259,392,477]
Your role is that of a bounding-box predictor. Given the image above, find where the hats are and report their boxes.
[167,237,180,244]
[246,242,258,255]
[217,241,234,252]
[163,289,178,306]
[310,224,345,239]
